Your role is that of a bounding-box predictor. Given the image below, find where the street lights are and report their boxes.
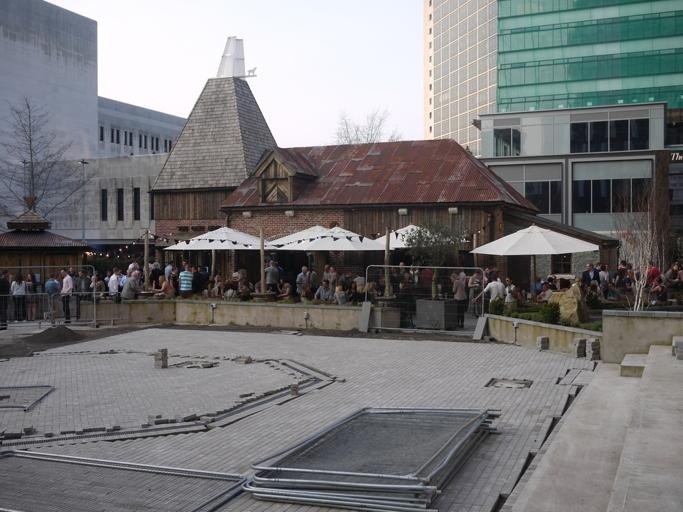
[22,160,28,214]
[79,158,89,246]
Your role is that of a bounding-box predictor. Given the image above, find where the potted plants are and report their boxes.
[400,222,477,330]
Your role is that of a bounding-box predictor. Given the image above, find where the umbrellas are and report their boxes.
[162,227,269,296]
[269,224,356,270]
[468,221,601,303]
[329,227,395,266]
[376,222,470,248]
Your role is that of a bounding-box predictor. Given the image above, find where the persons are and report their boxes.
[2,256,683,327]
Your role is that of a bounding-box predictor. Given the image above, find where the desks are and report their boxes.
[250,291,276,303]
[138,291,155,301]
[374,295,398,307]
